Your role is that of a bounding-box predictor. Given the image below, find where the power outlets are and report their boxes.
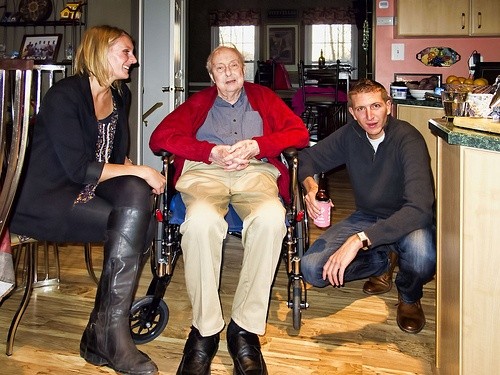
[391,43,405,61]
[469,56,484,74]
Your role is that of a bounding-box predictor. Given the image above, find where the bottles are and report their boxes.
[319,51,325,69]
[313,172,332,231]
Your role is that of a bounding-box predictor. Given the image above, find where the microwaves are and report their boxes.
[473,62,500,86]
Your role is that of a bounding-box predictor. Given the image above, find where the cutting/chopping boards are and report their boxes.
[452,116,500,135]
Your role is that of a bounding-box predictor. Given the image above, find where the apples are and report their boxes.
[446,75,489,91]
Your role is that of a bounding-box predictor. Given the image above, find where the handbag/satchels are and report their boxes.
[254,58,292,90]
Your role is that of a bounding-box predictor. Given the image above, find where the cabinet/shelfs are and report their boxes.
[0,0,87,66]
[394,0,500,39]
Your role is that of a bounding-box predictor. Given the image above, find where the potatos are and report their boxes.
[407,77,438,90]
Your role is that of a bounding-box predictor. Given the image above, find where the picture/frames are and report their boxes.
[18,33,63,63]
[266,25,299,72]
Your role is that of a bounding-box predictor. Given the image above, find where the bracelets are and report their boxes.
[356,230,370,250]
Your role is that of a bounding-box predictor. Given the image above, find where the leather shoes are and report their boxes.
[396,291,426,334]
[175,325,220,375]
[226,318,268,375]
[362,252,397,295]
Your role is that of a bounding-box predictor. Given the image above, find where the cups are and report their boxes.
[441,91,468,117]
[435,87,444,96]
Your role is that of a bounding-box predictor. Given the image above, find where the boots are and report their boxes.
[80,206,160,375]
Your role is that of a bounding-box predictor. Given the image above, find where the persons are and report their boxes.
[3,26,168,375]
[24,40,55,60]
[291,80,436,333]
[148,44,310,375]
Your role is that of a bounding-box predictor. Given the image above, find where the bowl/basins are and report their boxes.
[470,93,494,117]
[409,89,434,100]
[390,86,407,99]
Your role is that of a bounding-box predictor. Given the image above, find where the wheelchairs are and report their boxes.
[128,145,310,346]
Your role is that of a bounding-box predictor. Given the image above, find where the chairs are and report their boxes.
[256,59,348,143]
[0,58,101,357]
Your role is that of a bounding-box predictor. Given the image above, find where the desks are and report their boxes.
[274,87,346,103]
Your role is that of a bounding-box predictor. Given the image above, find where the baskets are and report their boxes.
[443,83,493,94]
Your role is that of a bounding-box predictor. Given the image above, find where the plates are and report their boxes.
[18,0,52,22]
[443,83,490,93]
